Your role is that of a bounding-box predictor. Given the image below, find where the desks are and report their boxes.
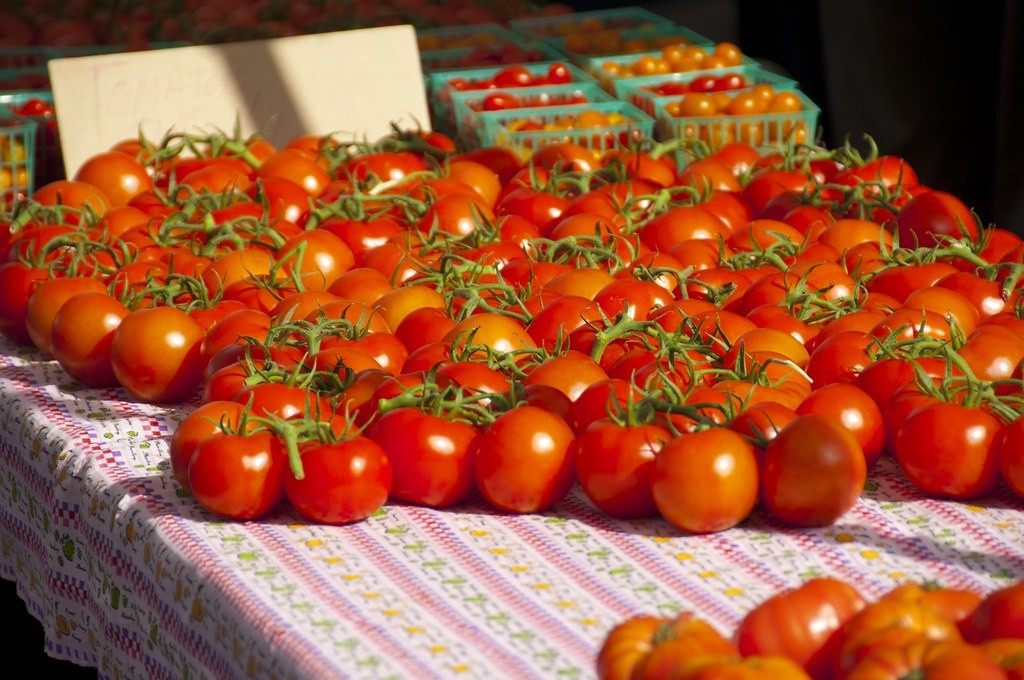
[0,330,1023,680]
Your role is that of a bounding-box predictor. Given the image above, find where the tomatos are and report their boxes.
[0,0,1024,680]
